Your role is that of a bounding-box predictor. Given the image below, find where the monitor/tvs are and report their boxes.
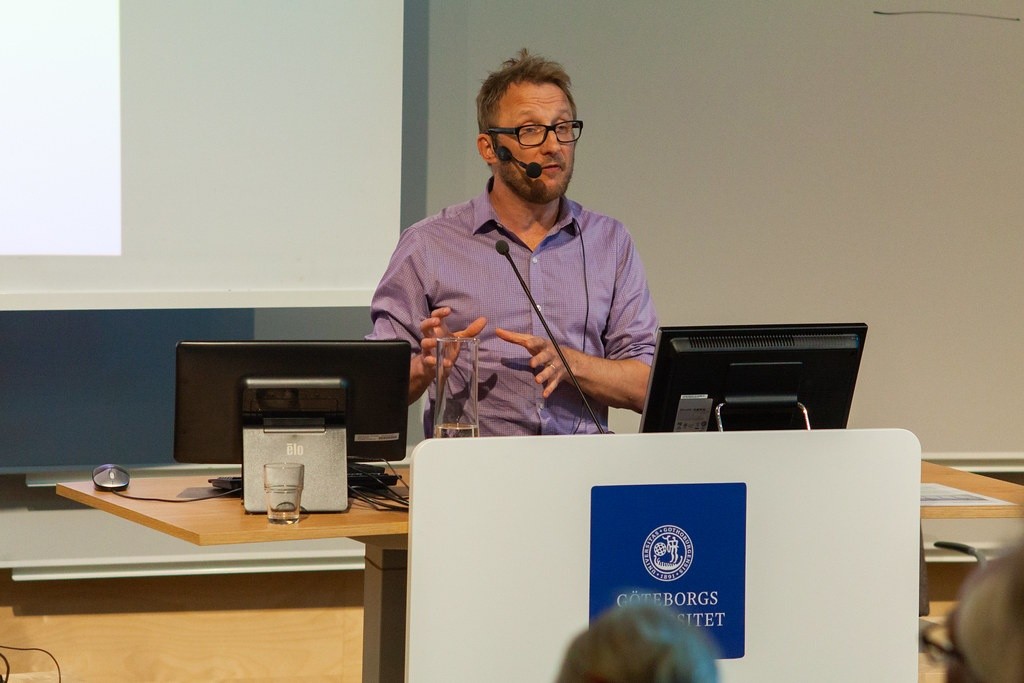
[635,320,869,435]
[172,338,410,523]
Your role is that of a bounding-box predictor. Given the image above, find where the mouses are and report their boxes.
[92,462,132,492]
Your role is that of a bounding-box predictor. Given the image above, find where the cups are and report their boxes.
[432,337,480,437]
[264,463,305,524]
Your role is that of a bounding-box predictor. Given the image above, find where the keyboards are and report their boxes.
[206,469,403,491]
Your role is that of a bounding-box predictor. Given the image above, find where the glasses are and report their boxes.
[923,624,962,663]
[484,120,584,146]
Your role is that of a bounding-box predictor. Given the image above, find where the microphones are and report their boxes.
[493,145,542,178]
[495,239,608,433]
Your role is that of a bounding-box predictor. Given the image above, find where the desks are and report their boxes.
[56,459,1023,683]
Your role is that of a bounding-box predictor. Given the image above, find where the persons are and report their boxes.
[364,50,662,438]
[919,549,1024,683]
[556,598,719,683]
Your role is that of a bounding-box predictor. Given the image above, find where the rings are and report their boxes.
[550,364,556,370]
